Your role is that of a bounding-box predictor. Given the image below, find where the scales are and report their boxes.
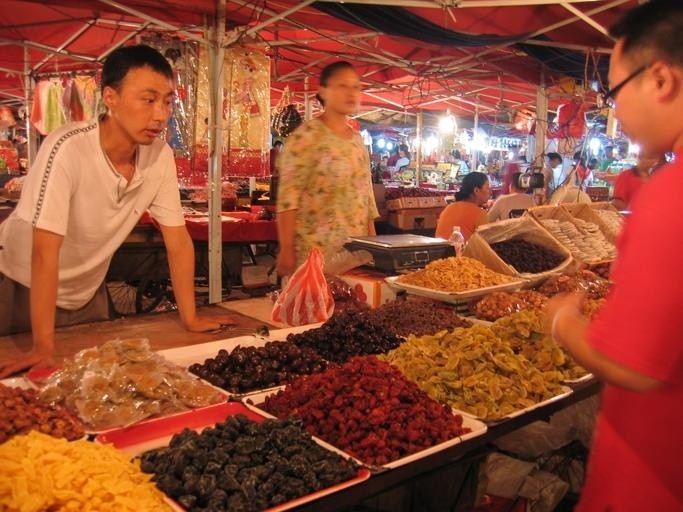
[342,233,456,276]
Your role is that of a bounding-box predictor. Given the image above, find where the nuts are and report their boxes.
[0,239,607,512]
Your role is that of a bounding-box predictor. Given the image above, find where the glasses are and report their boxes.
[601,65,649,108]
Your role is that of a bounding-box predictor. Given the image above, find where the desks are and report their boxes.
[140,198,278,243]
[0,297,683,508]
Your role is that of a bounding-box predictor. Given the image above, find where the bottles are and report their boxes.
[448,226,465,258]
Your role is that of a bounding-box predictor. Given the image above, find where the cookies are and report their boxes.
[540,208,624,262]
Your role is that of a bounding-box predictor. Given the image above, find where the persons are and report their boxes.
[0,45,239,379]
[387,139,667,241]
[551,0,683,512]
[270,141,282,172]
[276,61,381,278]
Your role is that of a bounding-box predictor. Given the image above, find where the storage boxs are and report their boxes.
[463,201,630,281]
[385,197,447,230]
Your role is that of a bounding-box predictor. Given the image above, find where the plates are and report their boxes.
[155,335,331,398]
[251,321,408,366]
[241,383,489,470]
[39,367,231,436]
[0,377,92,442]
[385,274,526,304]
[358,309,495,339]
[471,314,594,386]
[442,382,575,428]
[94,402,372,512]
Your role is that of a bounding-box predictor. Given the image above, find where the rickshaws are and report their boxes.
[104,243,282,320]
